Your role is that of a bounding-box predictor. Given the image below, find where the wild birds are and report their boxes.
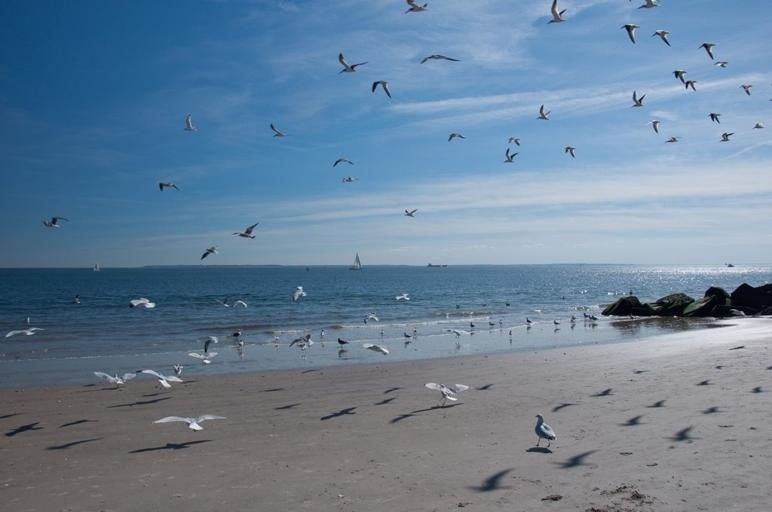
[404,206,419,219]
[199,222,259,261]
[182,113,195,135]
[40,214,69,230]
[532,413,556,448]
[159,180,180,191]
[3,324,44,342]
[267,123,288,141]
[151,413,229,434]
[326,0,767,185]
[91,280,599,405]
